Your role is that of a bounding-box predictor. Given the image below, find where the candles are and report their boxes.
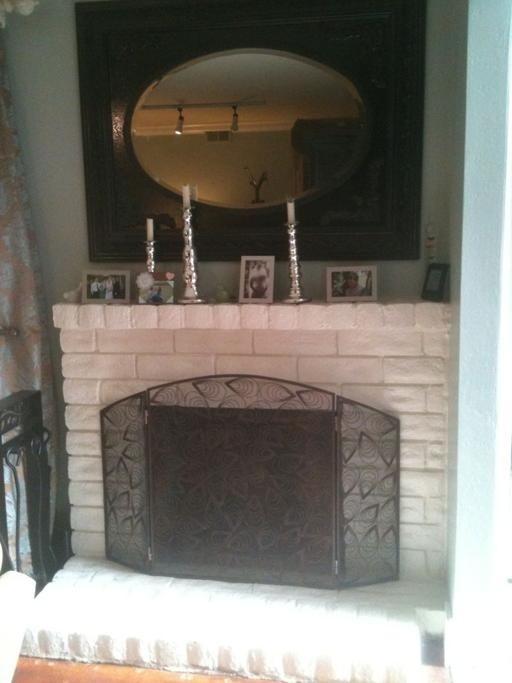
[286,198,295,223]
[182,183,191,209]
[146,217,154,242]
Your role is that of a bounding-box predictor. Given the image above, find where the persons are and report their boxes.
[91,276,114,298]
[342,273,366,296]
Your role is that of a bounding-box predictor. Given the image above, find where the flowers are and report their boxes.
[135,272,154,289]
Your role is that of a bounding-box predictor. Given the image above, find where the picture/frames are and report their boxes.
[80,269,131,305]
[237,255,276,304]
[421,262,449,301]
[326,265,378,302]
[138,281,175,305]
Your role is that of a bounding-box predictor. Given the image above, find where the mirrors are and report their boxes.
[73,2,424,263]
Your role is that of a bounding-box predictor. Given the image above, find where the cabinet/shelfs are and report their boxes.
[290,118,368,197]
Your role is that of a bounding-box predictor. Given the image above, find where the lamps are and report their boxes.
[173,106,186,137]
[230,104,239,131]
